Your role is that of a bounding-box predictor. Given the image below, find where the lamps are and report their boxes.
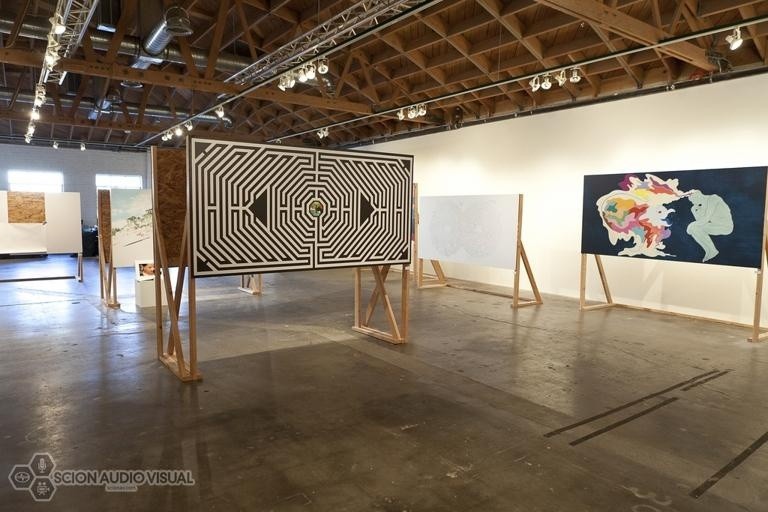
[52,141,60,150]
[528,68,582,94]
[724,27,744,51]
[316,127,330,139]
[80,142,87,152]
[277,58,329,92]
[214,107,224,119]
[396,104,427,122]
[44,16,66,72]
[184,121,193,132]
